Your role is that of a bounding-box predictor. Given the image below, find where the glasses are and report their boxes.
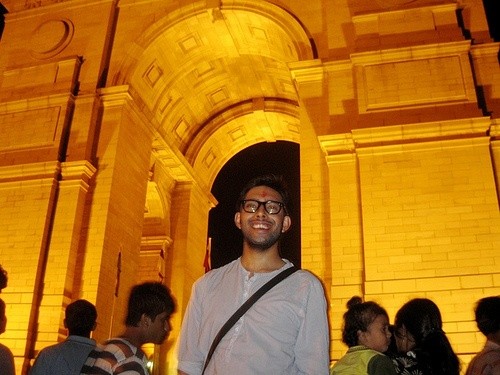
[240,199,285,215]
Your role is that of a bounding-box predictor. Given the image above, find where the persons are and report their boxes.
[175,173,330,375]
[0,263,178,375]
[331,293,500,375]
